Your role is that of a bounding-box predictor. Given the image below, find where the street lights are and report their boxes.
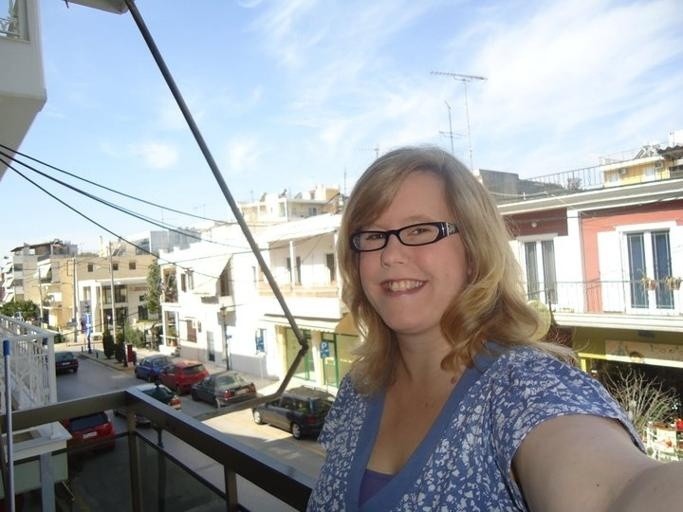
[149,382,169,510]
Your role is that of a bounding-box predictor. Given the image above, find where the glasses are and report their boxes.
[349,220,461,255]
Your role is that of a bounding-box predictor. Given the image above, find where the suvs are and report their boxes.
[254,385,337,440]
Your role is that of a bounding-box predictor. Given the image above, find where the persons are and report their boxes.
[305,147,683,512]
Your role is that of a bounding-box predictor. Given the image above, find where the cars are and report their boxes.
[54,349,78,376]
[134,354,174,382]
[156,360,208,395]
[111,382,182,425]
[61,411,115,458]
[191,370,256,407]
